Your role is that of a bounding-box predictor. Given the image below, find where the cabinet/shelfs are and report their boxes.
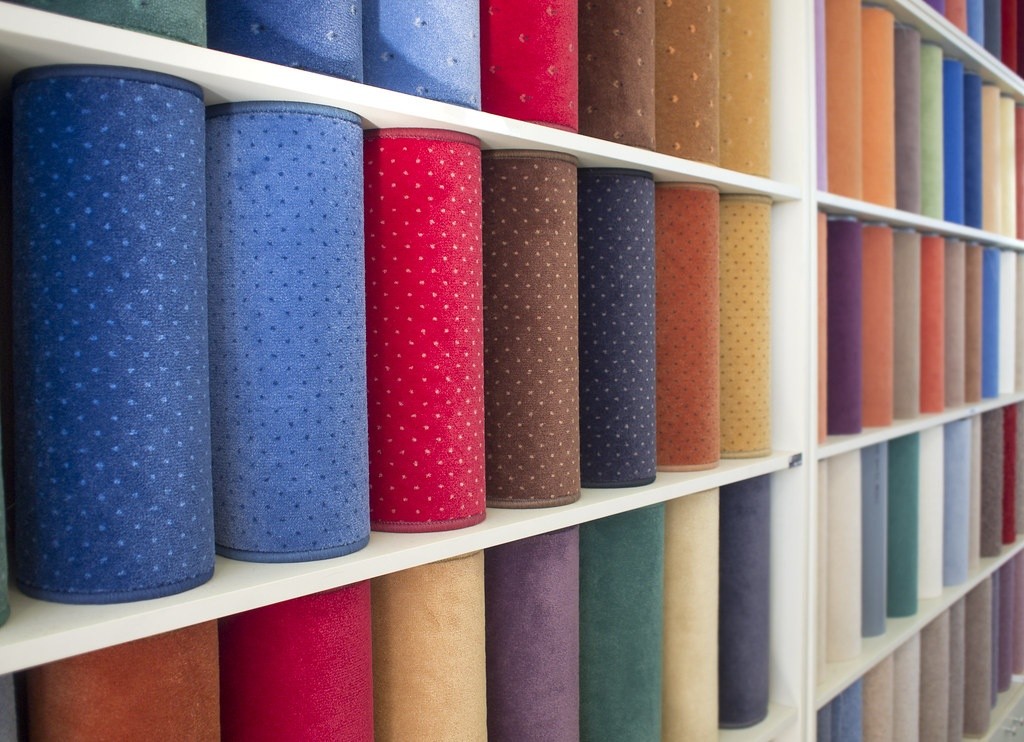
[0,0,1023,742]
